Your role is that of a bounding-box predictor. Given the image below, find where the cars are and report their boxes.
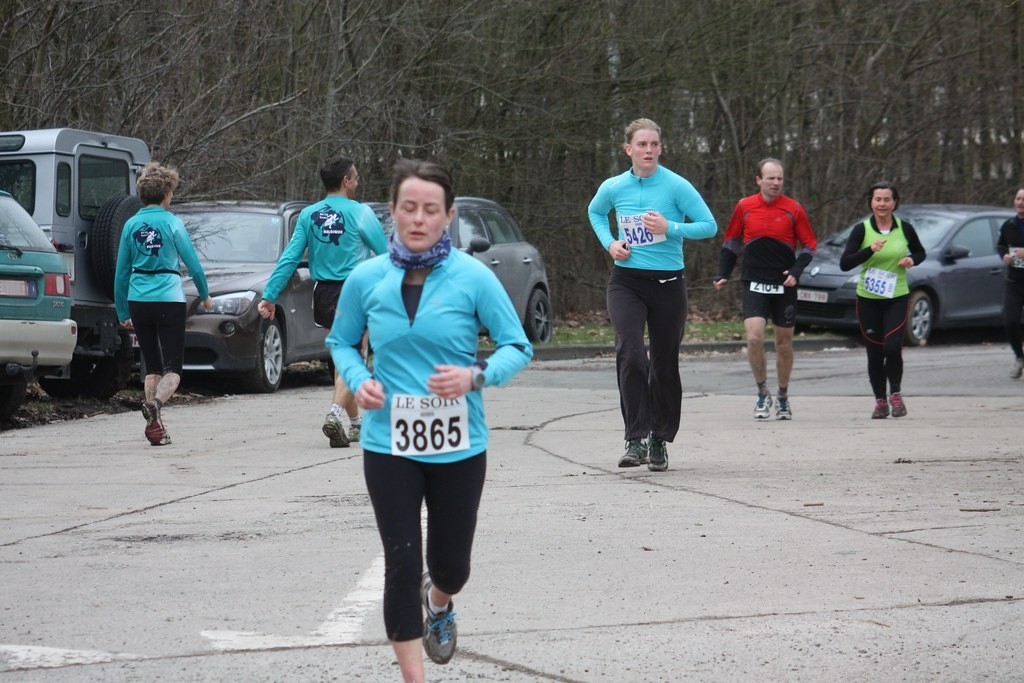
[0,190,78,421]
[792,202,1019,348]
[363,196,554,347]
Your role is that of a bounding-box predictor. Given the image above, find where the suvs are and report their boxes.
[129,199,370,395]
[0,128,156,402]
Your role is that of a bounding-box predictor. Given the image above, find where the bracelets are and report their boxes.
[470,365,485,391]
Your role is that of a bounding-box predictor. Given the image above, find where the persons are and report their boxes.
[587,117,718,471]
[114,161,214,446]
[324,157,535,683]
[839,181,927,418]
[258,156,390,448]
[997,186,1024,378]
[713,158,817,421]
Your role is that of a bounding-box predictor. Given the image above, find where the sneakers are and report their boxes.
[1009,358,1024,378]
[871,398,889,418]
[141,400,166,444]
[618,440,648,466]
[322,411,350,447]
[753,393,773,418]
[775,397,791,419]
[420,571,458,664]
[888,392,907,417]
[649,437,668,470]
[163,428,171,445]
[349,425,360,441]
